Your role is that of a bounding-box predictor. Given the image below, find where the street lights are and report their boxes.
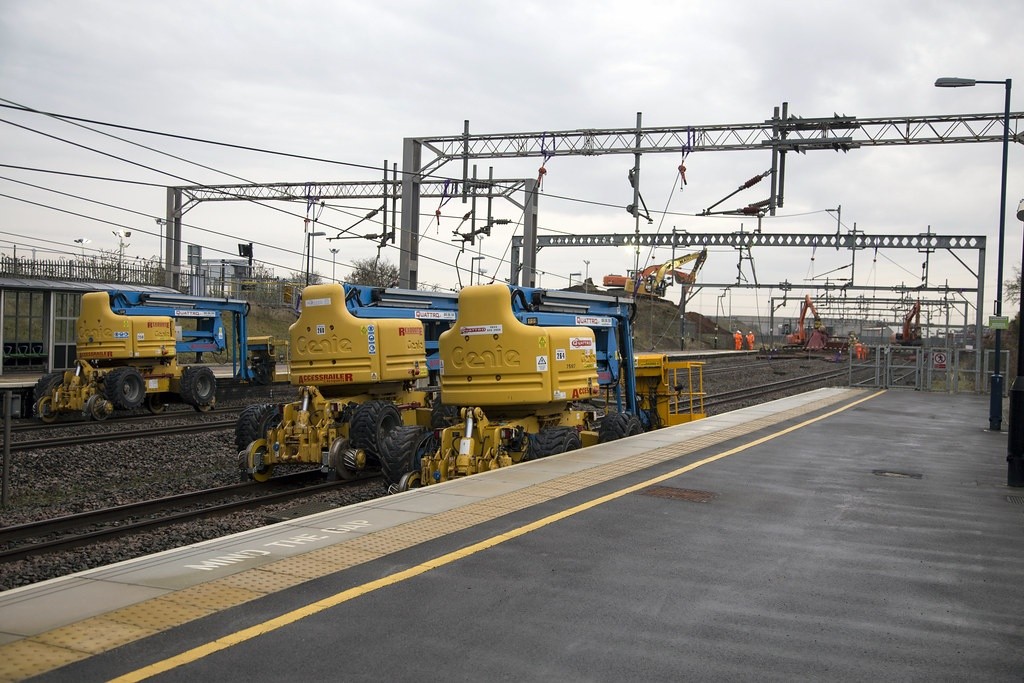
[585,260,590,294]
[569,273,581,288]
[155,217,167,267]
[330,248,340,283]
[113,229,131,282]
[470,257,485,286]
[306,232,326,286]
[714,295,725,349]
[934,78,1012,431]
[74,238,93,277]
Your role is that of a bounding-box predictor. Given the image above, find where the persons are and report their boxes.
[854,341,868,363]
[733,330,754,350]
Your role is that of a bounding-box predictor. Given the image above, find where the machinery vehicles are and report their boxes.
[603,248,708,307]
[756,294,850,363]
[867,301,931,361]
[381,283,706,494]
[26,289,291,424]
[233,283,458,484]
